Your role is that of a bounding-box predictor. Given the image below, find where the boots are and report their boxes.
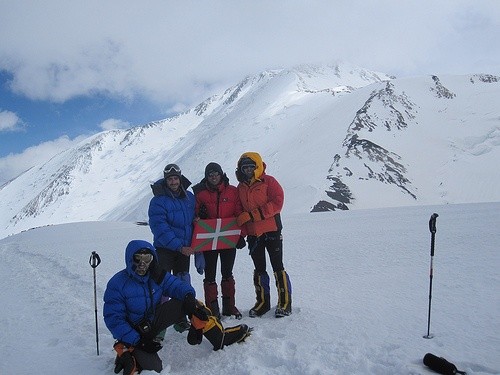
[113,339,144,375]
[202,316,253,351]
[220,278,243,320]
[202,279,221,320]
[249,272,271,319]
[274,270,293,317]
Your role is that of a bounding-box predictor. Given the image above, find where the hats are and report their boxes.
[240,158,257,178]
[164,164,182,180]
[204,161,224,178]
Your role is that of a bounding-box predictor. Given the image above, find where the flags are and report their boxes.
[191,217,242,250]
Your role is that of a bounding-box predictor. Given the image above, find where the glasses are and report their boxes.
[167,174,178,180]
[208,172,221,177]
[134,254,153,265]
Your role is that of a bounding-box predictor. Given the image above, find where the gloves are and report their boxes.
[194,252,205,275]
[182,294,210,322]
[137,336,164,354]
[237,206,266,227]
[236,237,247,249]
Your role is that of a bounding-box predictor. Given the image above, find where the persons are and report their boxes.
[191,162,247,321]
[103,240,250,374]
[148,163,196,332]
[234,151,292,317]
